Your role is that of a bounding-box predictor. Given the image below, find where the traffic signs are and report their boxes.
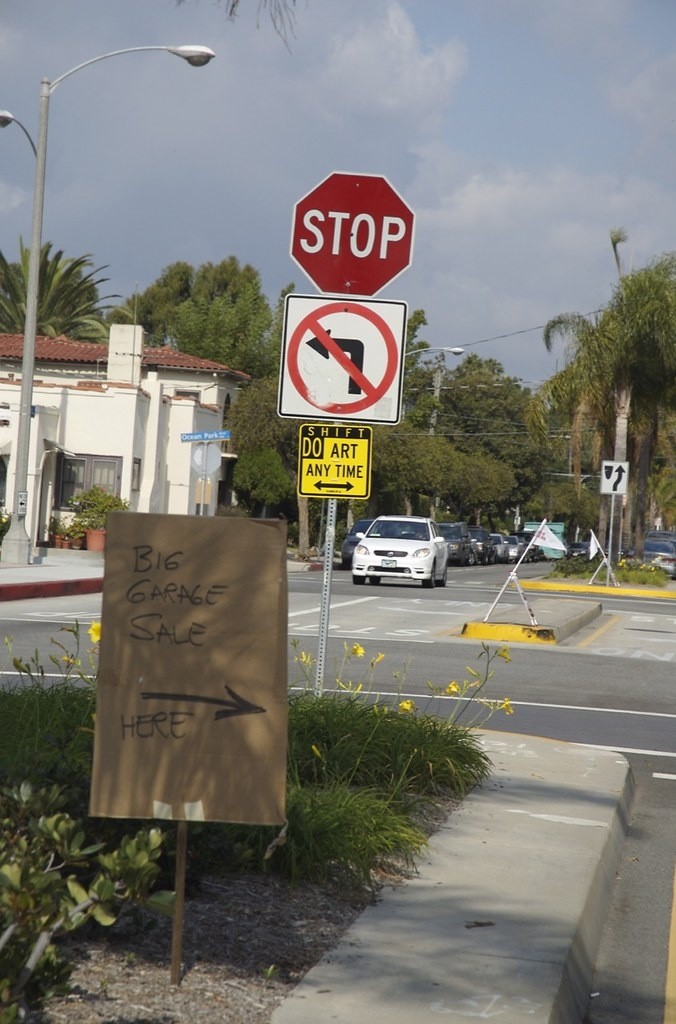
[297,423,375,501]
[83,501,294,828]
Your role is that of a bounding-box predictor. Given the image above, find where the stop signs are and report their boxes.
[289,169,416,299]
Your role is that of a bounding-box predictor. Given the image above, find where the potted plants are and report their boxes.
[47,485,131,552]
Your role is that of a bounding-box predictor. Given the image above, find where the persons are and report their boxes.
[414,528,426,540]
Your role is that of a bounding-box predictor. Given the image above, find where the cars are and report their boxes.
[436,520,625,565]
[340,518,378,571]
[643,529,676,581]
[351,515,449,589]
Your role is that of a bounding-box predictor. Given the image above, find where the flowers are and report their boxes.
[0,505,13,547]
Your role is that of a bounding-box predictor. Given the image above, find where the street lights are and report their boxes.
[0,43,221,565]
[345,346,466,535]
